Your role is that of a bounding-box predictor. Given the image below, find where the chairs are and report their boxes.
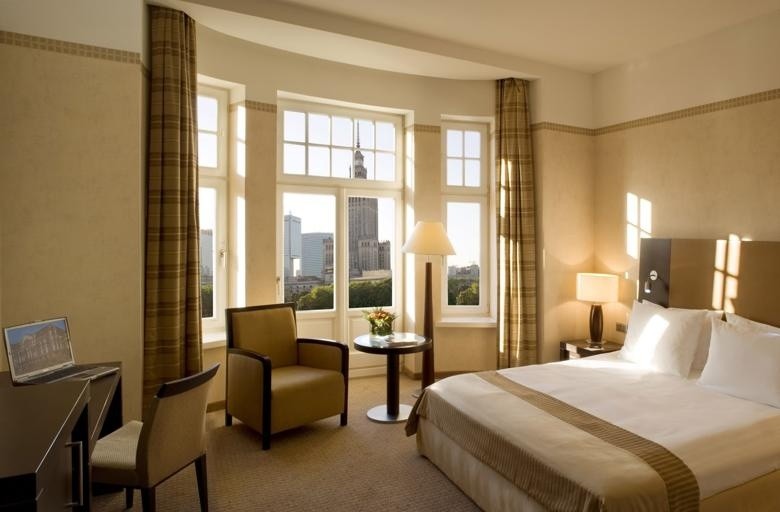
[83,361,221,510]
[224,301,356,449]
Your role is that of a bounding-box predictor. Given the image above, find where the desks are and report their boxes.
[0,359,129,511]
[348,330,437,427]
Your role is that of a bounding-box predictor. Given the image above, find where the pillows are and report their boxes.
[641,297,724,376]
[723,307,780,335]
[618,298,710,382]
[691,313,780,409]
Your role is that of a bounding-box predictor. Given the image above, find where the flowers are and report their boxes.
[361,303,401,335]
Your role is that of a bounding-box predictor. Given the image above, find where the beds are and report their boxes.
[402,238,780,511]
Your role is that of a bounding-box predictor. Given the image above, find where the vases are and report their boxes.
[365,319,397,342]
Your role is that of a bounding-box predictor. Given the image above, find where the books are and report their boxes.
[387,336,418,346]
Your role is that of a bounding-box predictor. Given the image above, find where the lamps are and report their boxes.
[575,271,619,348]
[399,217,459,401]
[643,266,660,294]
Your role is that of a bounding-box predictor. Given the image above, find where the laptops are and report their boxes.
[1,316,120,385]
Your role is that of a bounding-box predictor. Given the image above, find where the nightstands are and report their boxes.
[556,338,618,361]
